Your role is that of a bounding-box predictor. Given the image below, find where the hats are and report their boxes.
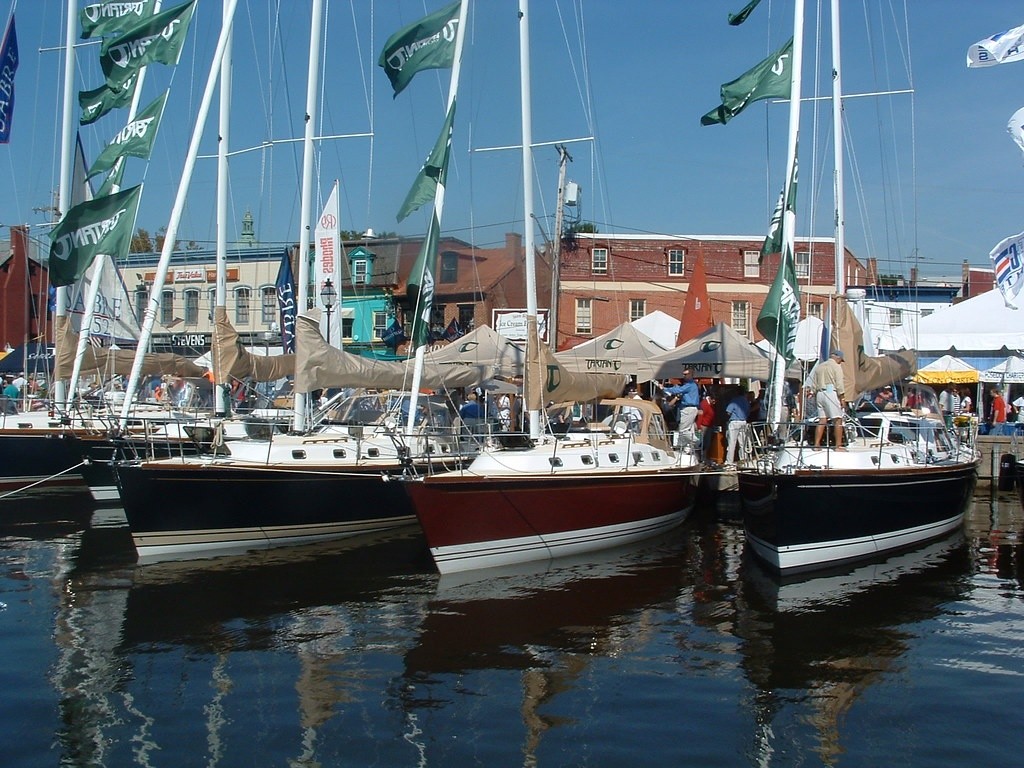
[832,350,845,362]
[884,386,891,388]
[626,387,638,392]
[19,372,24,375]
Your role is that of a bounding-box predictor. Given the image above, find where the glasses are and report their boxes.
[683,374,689,376]
[953,390,957,393]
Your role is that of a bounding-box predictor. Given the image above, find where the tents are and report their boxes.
[52,286,1024,423]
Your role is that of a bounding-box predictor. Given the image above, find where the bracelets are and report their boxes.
[841,397,846,401]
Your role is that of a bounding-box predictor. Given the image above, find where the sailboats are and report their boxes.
[728,0,987,564]
[395,0,702,577]
[0,1,416,559]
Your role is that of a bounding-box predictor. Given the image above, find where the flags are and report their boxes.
[989,232,1024,310]
[0,12,20,143]
[728,0,761,27]
[380,319,407,348]
[442,317,465,343]
[396,97,457,354]
[967,25,1024,68]
[378,0,462,101]
[1007,107,1024,153]
[45,0,196,287]
[275,248,298,355]
[756,132,802,370]
[701,36,793,127]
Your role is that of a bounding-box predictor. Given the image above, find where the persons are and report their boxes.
[0,350,1024,469]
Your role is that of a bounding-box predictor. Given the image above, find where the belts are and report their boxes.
[686,405,698,407]
